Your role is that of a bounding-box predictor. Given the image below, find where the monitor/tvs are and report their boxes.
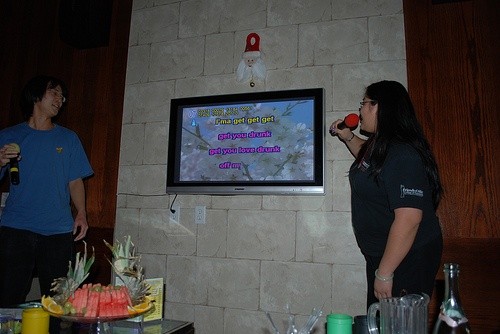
[165,87,325,196]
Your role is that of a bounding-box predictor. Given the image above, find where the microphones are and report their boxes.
[330,113,359,136]
[7,143,21,185]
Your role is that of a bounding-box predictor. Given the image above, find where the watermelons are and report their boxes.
[67,282,133,317]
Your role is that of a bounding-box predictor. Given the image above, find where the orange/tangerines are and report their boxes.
[127,303,152,313]
[42,294,64,314]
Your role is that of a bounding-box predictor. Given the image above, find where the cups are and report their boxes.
[327,313,353,334]
[366,294,428,334]
[21,309,50,334]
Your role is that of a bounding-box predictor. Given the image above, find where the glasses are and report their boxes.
[47,88,65,102]
[360,100,376,107]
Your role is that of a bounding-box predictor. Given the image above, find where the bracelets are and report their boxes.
[344,132,354,143]
[374,269,395,282]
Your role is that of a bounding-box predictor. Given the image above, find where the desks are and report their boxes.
[0,316,196,334]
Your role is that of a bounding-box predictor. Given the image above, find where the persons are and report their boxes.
[0,75,95,309]
[329,80,444,334]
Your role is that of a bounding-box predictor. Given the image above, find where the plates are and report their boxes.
[41,296,155,323]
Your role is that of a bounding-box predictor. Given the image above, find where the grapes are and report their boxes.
[62,302,76,315]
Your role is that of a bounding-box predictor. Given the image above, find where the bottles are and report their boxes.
[354,315,378,334]
[434,264,471,334]
